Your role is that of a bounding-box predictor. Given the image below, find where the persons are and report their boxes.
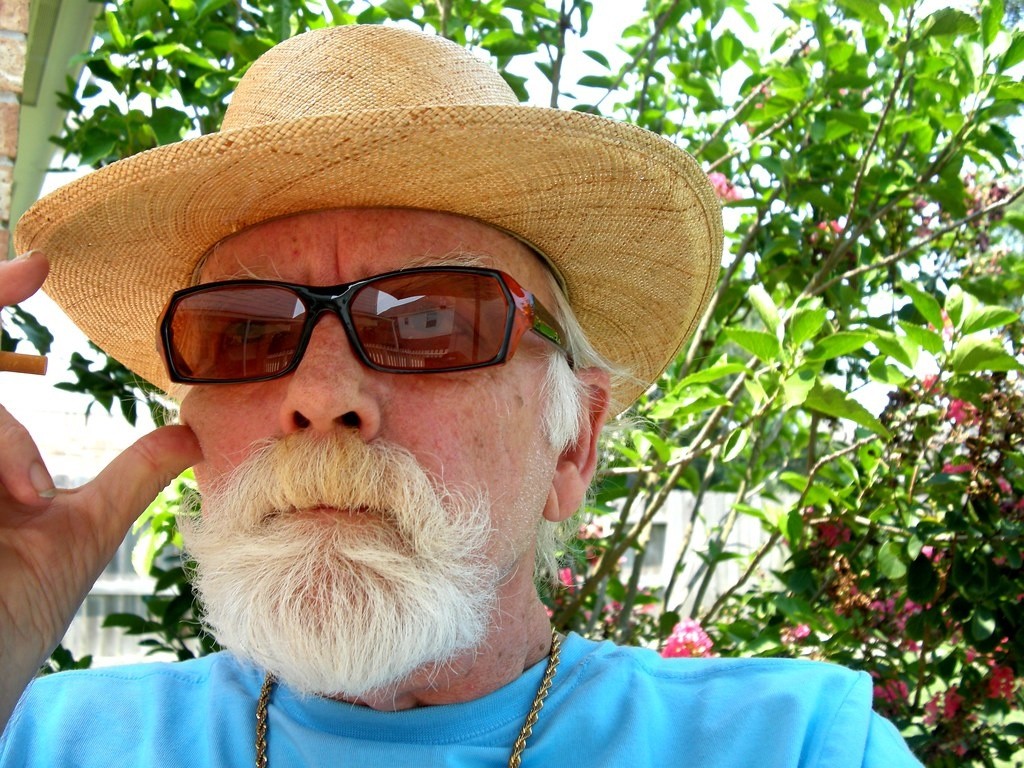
[0,25,925,768]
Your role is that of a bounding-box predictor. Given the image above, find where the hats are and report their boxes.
[12,26,723,427]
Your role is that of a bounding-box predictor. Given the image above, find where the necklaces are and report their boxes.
[255,625,561,768]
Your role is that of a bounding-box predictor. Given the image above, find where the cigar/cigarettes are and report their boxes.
[0,350,48,376]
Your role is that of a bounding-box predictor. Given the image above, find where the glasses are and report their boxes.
[153,264,574,384]
